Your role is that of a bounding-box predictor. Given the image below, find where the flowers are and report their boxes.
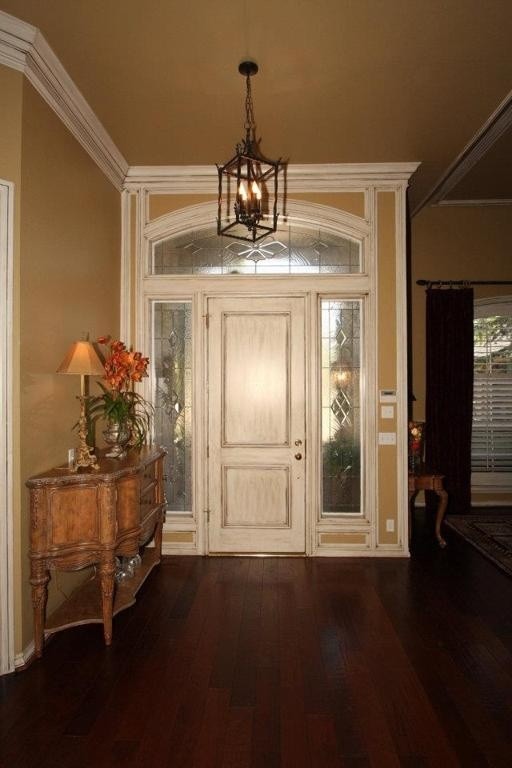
[72,334,162,455]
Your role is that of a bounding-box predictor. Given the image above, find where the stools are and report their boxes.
[408,468,451,548]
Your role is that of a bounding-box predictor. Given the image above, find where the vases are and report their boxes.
[103,421,131,458]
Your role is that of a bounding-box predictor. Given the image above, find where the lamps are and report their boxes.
[409,421,427,474]
[215,60,291,243]
[58,341,110,473]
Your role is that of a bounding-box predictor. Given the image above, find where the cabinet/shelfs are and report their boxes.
[24,443,175,659]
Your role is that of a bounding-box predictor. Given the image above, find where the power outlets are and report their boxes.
[68,448,74,465]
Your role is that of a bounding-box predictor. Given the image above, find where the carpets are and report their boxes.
[444,512,512,580]
